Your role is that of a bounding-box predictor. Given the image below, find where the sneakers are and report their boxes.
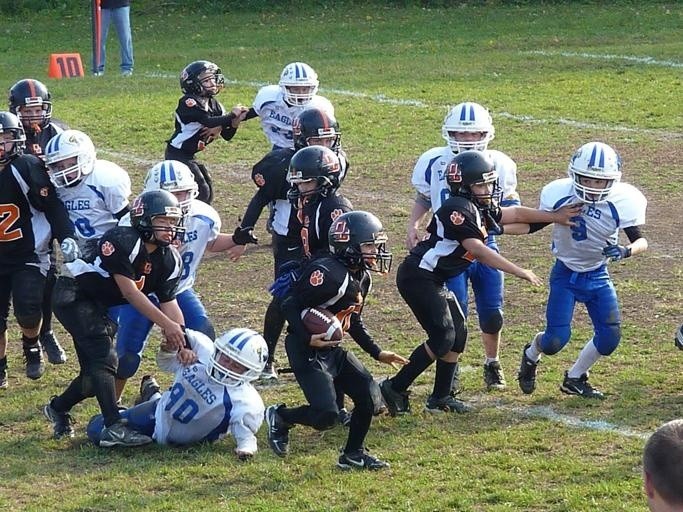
[99,418,152,447]
[337,446,391,472]
[22,339,46,380]
[424,396,476,415]
[0,360,8,391]
[43,394,76,440]
[134,374,160,406]
[40,331,67,365]
[558,369,605,399]
[483,359,507,392]
[263,402,290,459]
[518,342,541,394]
[378,377,412,417]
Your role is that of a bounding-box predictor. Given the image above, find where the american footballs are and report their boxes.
[301,305,343,341]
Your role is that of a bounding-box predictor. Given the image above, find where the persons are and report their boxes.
[378,152,583,416]
[198,61,336,151]
[406,102,522,390]
[165,59,249,205]
[485,142,647,400]
[0,88,270,470]
[643,418,683,512]
[229,108,411,471]
[91,0,134,78]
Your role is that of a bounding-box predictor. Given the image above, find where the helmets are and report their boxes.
[0,112,26,135]
[179,60,220,97]
[445,151,497,201]
[278,61,319,87]
[292,107,340,151]
[8,78,51,117]
[289,145,341,198]
[214,327,269,373]
[142,159,194,193]
[441,101,493,133]
[326,209,388,266]
[569,141,622,178]
[130,189,181,243]
[44,129,96,176]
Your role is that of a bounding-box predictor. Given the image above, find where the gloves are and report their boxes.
[232,226,259,247]
[601,239,632,261]
[60,237,83,263]
[480,210,504,236]
